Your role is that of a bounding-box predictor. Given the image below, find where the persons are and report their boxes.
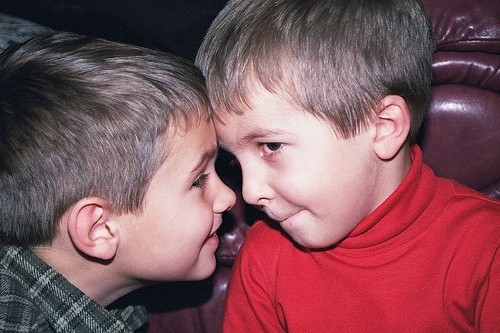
[194,0,500,333]
[0,29,236,333]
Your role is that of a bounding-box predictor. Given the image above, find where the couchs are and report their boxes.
[148,0,500,333]
